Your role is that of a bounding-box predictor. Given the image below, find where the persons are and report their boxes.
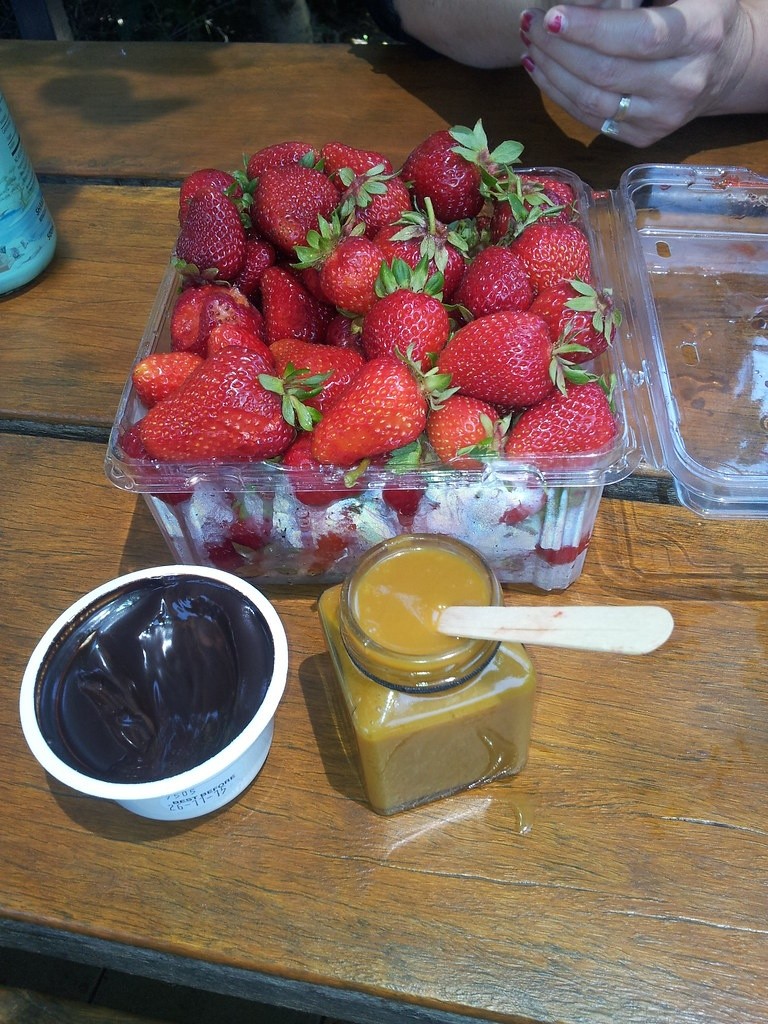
[370,0,768,153]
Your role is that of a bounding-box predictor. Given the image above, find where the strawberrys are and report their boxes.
[113,118,619,591]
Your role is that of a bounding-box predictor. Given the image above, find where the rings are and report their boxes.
[613,95,631,121]
[601,118,618,138]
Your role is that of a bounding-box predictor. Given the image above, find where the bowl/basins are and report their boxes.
[17,564,288,821]
[98,157,768,591]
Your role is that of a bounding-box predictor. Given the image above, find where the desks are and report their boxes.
[0,35,767,1024]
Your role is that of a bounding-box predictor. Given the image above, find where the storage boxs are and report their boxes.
[105,160,767,590]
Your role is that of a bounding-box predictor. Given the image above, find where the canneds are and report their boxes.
[312,532,539,815]
[0,87,56,300]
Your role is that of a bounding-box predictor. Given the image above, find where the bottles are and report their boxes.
[1,89,57,297]
[318,534,538,818]
